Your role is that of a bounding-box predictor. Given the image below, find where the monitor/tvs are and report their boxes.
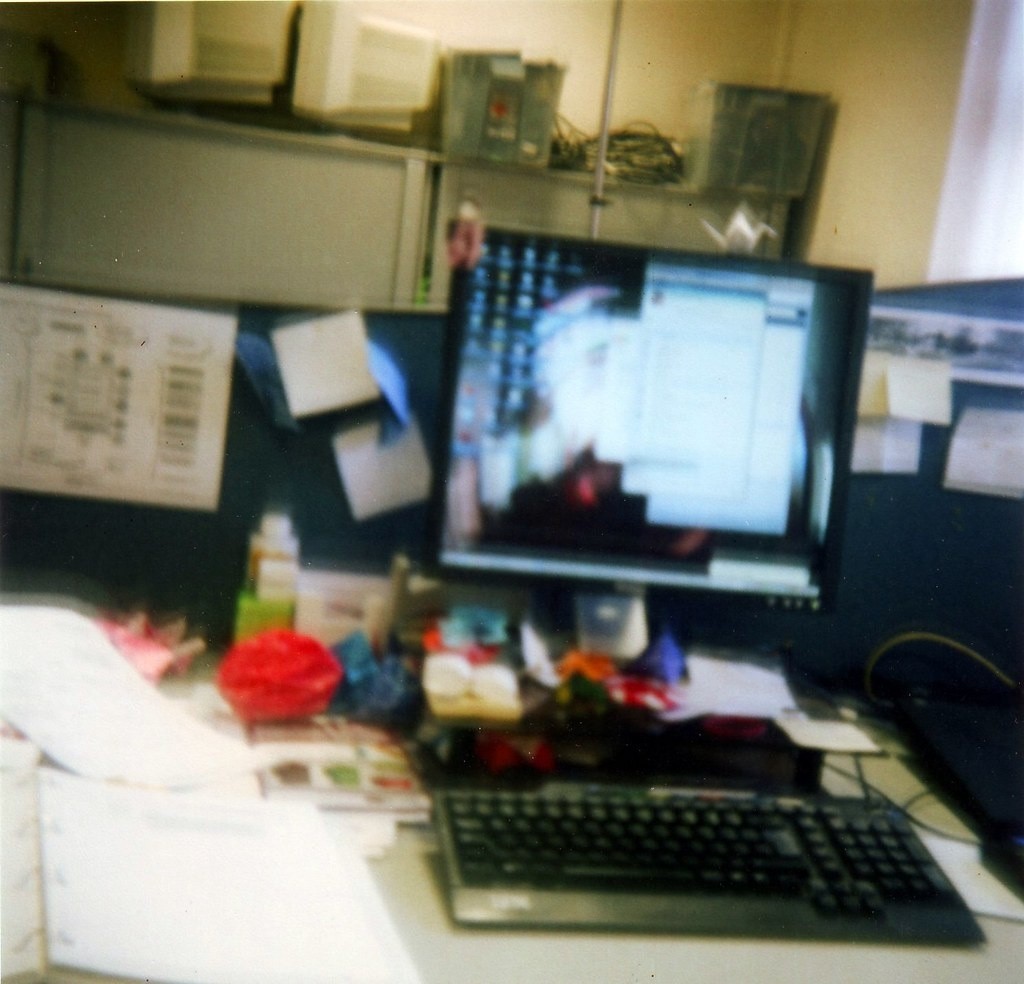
[416,227,876,681]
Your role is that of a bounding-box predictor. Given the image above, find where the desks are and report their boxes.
[0,573,1024,984]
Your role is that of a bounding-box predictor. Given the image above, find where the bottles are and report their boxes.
[244,514,301,602]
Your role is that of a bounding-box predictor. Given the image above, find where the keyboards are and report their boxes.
[429,778,989,951]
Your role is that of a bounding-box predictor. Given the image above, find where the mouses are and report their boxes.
[980,830,1024,901]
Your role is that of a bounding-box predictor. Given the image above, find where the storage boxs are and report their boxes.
[446,48,569,167]
[707,82,830,196]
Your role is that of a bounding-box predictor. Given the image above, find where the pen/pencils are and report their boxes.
[375,553,413,651]
